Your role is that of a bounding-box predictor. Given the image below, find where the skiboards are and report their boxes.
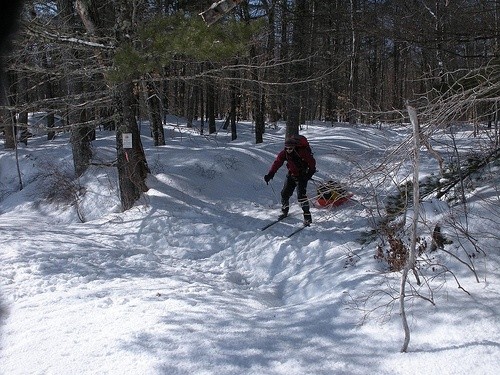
[261,214,312,237]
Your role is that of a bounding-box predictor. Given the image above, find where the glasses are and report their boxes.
[285,148,293,150]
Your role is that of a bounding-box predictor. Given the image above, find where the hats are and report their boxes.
[284,140,294,147]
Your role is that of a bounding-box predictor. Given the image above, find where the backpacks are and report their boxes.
[285,135,311,164]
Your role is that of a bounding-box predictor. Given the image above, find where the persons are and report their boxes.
[264,138,316,225]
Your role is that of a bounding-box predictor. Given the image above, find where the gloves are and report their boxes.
[302,174,310,182]
[264,175,270,183]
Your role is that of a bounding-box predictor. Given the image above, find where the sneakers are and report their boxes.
[304,215,311,225]
[278,211,288,219]
